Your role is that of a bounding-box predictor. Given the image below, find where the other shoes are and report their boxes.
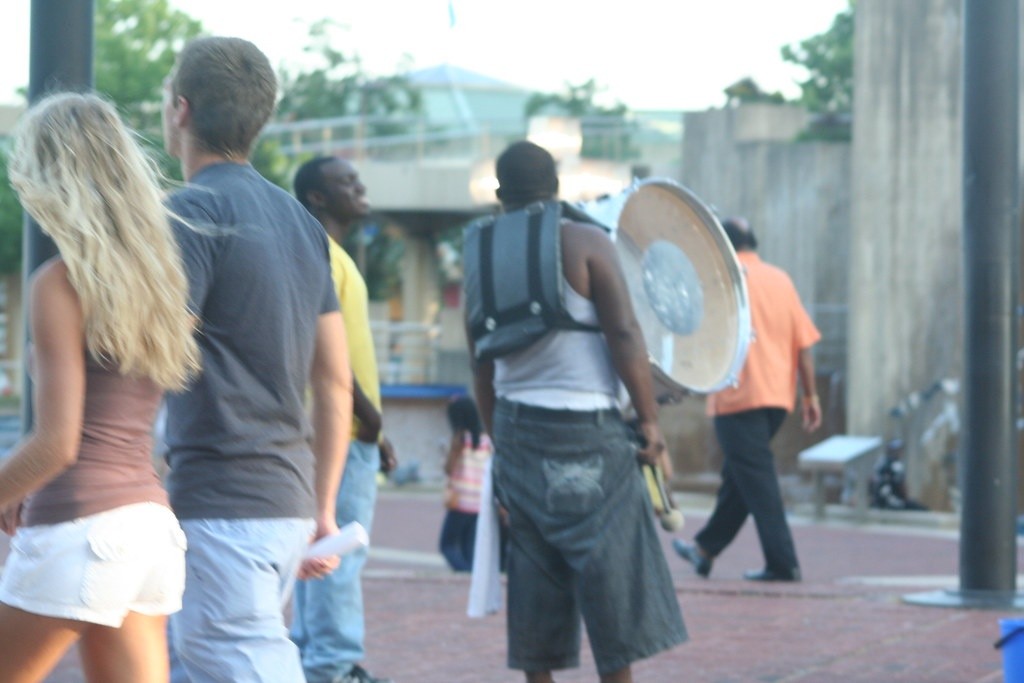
[744,567,801,582]
[338,665,392,683]
[672,537,711,576]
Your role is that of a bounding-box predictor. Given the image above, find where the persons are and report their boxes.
[0,92,201,683]
[290,157,397,683]
[439,396,491,572]
[463,141,690,683]
[673,216,821,581]
[157,38,353,683]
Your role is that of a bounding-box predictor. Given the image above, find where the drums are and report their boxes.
[574,176,754,402]
